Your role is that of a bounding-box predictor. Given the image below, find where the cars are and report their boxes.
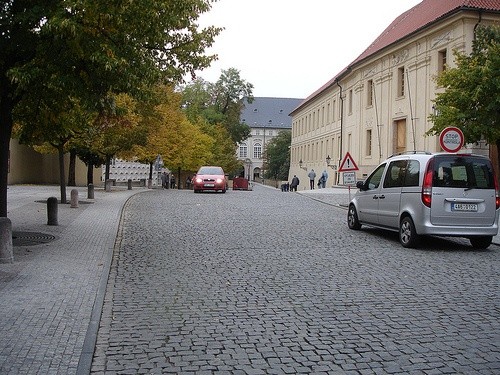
[193,166,229,193]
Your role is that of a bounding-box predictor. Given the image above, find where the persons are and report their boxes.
[280,174,299,192]
[161,171,175,189]
[186,172,196,190]
[318,170,328,189]
[307,168,316,190]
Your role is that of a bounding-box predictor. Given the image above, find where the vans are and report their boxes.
[347,150,500,249]
